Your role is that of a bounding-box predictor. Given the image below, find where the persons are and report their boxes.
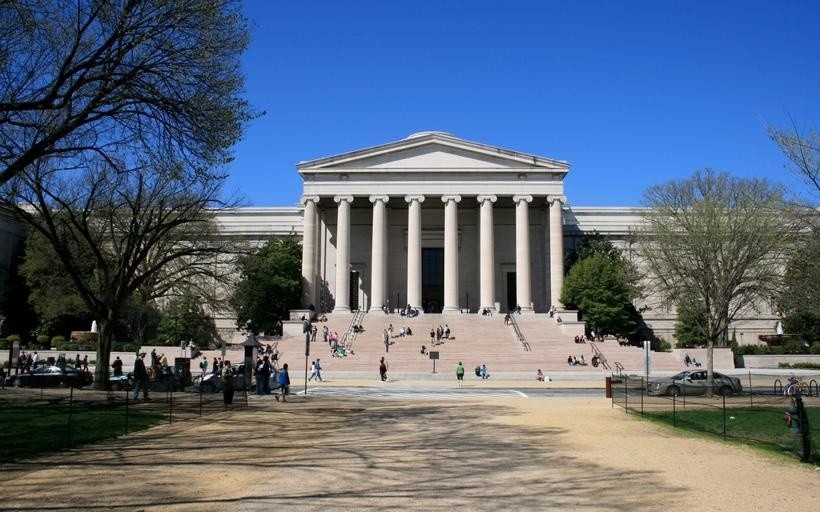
[456,361,464,388]
[308,359,322,381]
[536,369,552,382]
[684,354,700,367]
[19,350,290,410]
[430,324,450,346]
[311,325,338,348]
[383,323,412,345]
[302,314,327,334]
[516,304,521,315]
[382,301,394,314]
[400,304,418,318]
[379,357,388,381]
[549,305,556,317]
[504,313,511,326]
[574,327,630,346]
[475,364,490,379]
[567,354,600,367]
[482,308,492,316]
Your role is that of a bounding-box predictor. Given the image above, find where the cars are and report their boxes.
[648,368,743,397]
[5,365,93,389]
[108,366,194,392]
[193,361,284,395]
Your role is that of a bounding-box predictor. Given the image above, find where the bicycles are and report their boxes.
[782,375,810,398]
